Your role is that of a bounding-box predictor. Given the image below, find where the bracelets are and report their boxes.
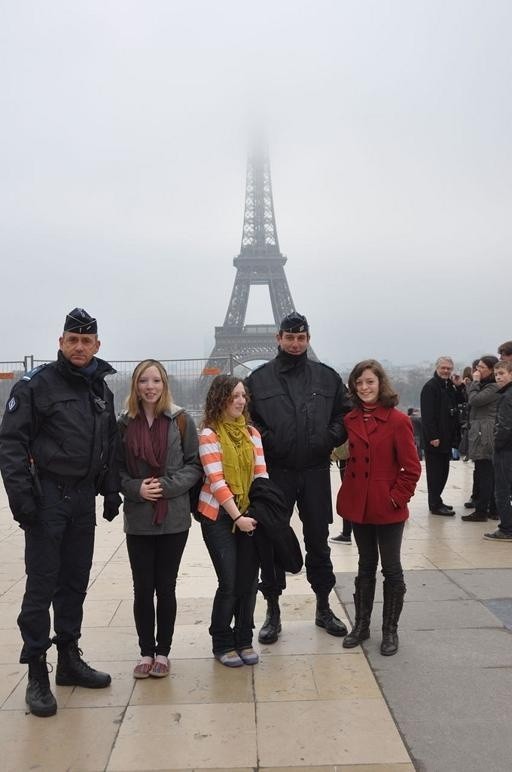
[232,513,245,522]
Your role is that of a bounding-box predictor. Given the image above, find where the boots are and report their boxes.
[56,638,111,689]
[316,597,347,634]
[26,655,57,717]
[382,581,405,655]
[258,603,281,643]
[343,577,376,648]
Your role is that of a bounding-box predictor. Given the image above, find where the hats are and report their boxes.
[280,312,308,332]
[64,309,97,334]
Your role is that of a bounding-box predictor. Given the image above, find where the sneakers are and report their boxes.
[330,535,352,544]
[484,525,512,541]
[134,655,170,678]
[462,498,498,521]
[214,648,259,667]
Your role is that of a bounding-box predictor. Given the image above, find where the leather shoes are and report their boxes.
[433,503,455,515]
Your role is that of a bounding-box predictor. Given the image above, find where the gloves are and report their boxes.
[102,492,122,521]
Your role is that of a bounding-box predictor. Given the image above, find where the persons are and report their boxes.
[1,307,122,718]
[114,360,203,680]
[244,309,354,645]
[421,356,460,518]
[452,340,512,539]
[335,359,424,655]
[197,372,270,667]
[331,436,352,546]
